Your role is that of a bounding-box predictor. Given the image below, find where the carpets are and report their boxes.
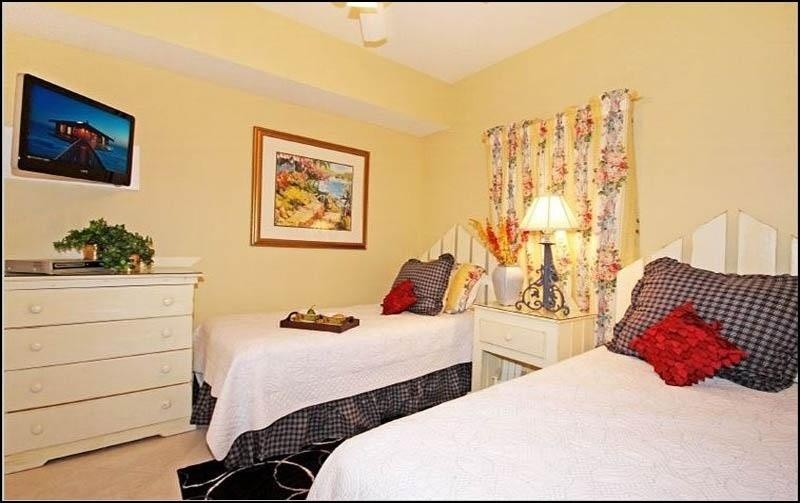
[176,437,344,500]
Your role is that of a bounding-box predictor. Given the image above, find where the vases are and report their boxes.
[492,263,524,306]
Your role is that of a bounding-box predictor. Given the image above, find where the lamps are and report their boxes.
[344,2,388,42]
[516,194,581,316]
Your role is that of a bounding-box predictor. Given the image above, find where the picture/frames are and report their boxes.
[250,125,370,250]
[10,71,136,187]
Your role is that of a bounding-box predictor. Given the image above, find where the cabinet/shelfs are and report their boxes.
[1,267,203,476]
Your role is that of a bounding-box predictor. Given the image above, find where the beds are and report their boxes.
[307,211,798,502]
[189,224,501,471]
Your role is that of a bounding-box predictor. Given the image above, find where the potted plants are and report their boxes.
[52,218,154,273]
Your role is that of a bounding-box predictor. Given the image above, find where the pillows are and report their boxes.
[381,280,419,315]
[628,303,751,387]
[390,253,454,316]
[444,261,486,313]
[604,256,798,393]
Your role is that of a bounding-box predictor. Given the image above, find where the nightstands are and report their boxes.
[468,301,597,392]
